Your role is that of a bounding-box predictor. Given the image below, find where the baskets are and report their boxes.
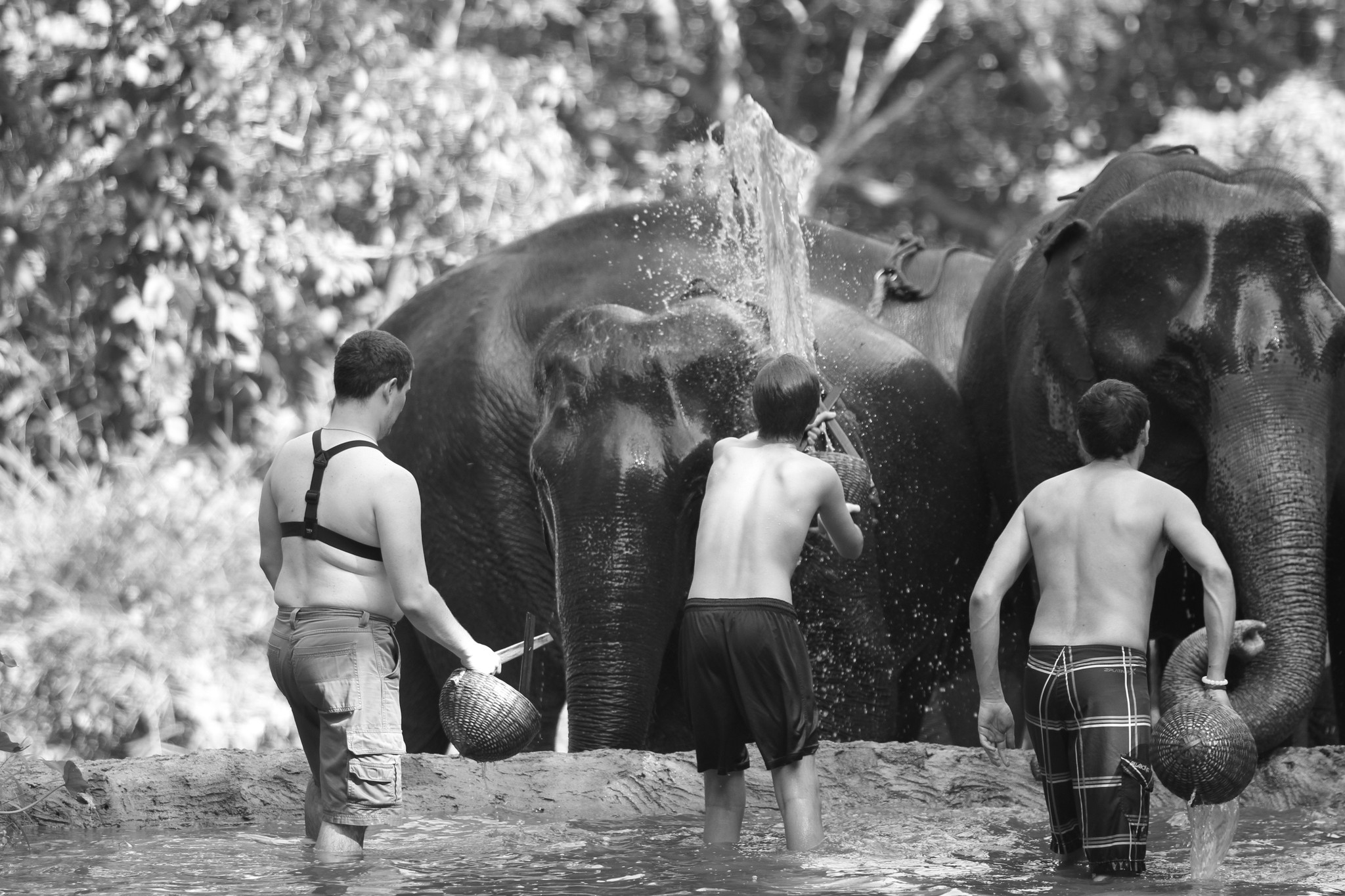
[1150,699,1259,805]
[799,380,871,511]
[438,612,555,762]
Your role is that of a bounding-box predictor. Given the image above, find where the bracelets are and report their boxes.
[1201,676,1228,691]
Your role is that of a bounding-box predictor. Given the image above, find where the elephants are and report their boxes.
[374,201,992,754]
[960,143,1344,766]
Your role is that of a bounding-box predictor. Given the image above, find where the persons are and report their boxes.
[258,331,502,871]
[969,381,1237,883]
[678,355,864,857]
